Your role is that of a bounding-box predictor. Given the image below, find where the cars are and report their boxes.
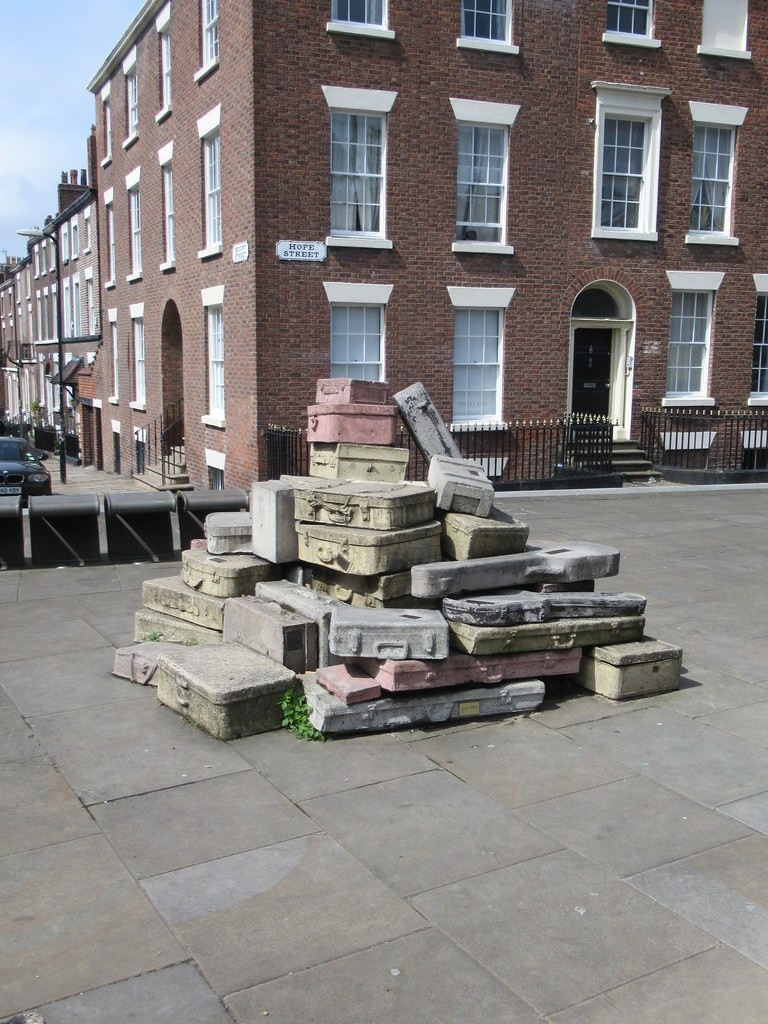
[0,436,52,508]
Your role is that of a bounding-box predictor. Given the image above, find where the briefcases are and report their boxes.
[111,378,683,741]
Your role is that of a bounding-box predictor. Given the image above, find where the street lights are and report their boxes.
[16,228,68,484]
